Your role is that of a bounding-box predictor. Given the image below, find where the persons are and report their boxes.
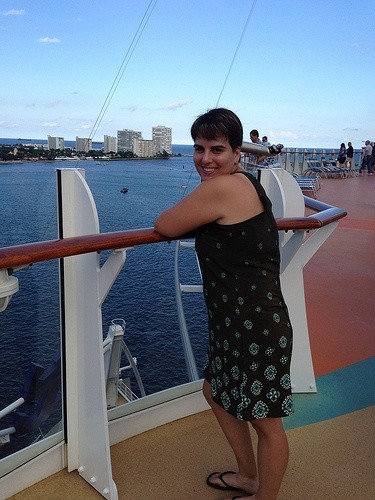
[244,129,276,171]
[154,108,295,500]
[356,140,375,176]
[337,142,354,179]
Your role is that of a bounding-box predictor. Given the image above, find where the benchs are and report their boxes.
[289,161,354,198]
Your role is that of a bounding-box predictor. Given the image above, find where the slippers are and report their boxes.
[206,472,257,496]
[226,494,256,500]
